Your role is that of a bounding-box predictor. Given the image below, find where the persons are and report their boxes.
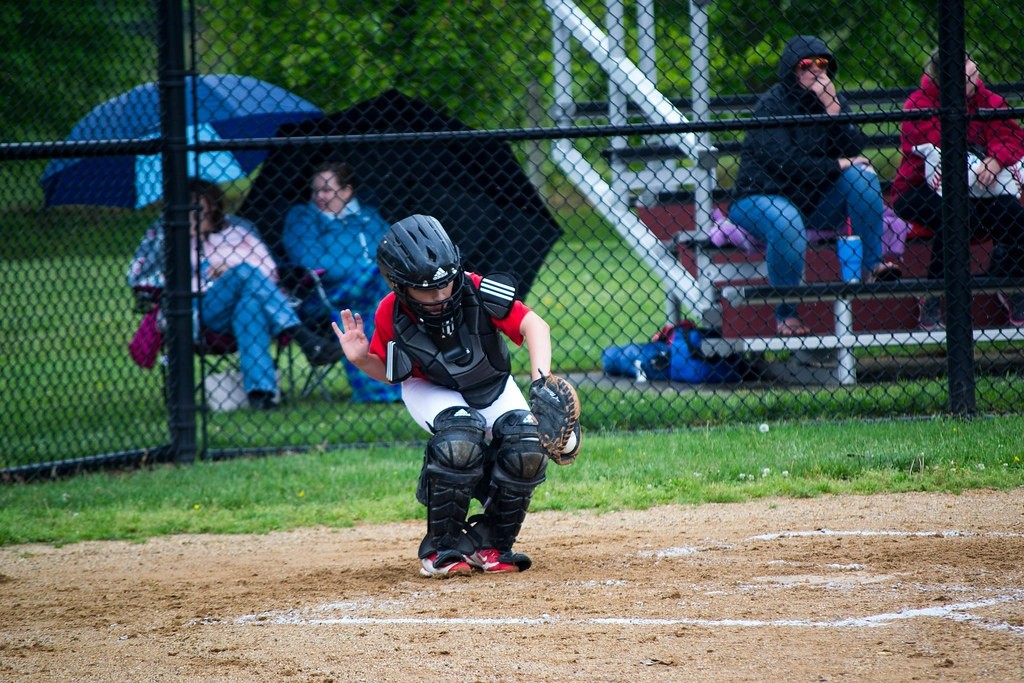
[283,162,403,404]
[889,47,1023,333]
[122,177,344,412]
[332,213,552,580]
[726,34,903,337]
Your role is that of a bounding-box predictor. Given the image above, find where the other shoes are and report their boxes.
[250,395,278,411]
[303,338,344,366]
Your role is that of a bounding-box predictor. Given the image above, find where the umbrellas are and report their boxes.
[37,73,329,214]
[235,86,566,304]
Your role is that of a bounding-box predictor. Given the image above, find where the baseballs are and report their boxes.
[561,431,577,455]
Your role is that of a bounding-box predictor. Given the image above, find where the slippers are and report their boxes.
[867,263,902,281]
[776,323,808,337]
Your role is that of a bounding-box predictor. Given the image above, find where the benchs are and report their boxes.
[552,80,1024,386]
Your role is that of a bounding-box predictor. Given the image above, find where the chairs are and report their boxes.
[127,266,341,403]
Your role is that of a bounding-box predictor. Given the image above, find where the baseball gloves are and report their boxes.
[529,375,583,466]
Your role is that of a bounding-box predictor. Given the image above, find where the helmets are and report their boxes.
[377,214,464,342]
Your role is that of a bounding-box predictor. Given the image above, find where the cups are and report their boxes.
[837,235,864,285]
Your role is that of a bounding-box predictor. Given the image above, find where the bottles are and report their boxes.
[199,256,217,287]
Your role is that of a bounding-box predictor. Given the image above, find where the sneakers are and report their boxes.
[463,549,519,574]
[420,551,471,579]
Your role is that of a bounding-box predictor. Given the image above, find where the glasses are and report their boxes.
[798,57,829,70]
